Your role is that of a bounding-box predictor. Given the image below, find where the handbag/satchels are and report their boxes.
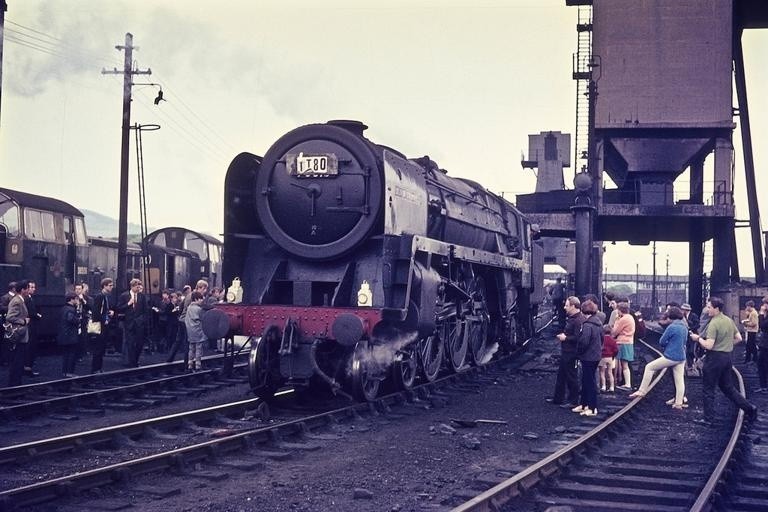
[3,322,27,344]
[694,336,704,358]
[88,320,101,335]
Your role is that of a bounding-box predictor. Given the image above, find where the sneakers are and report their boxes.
[545,384,688,416]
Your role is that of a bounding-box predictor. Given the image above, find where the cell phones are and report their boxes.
[688,329,693,336]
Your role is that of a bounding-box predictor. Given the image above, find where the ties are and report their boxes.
[134,293,137,312]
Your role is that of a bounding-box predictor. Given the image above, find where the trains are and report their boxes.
[0,188,223,343]
[201,120,546,404]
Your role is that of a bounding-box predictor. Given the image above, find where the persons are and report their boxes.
[548,277,766,426]
[1,277,221,386]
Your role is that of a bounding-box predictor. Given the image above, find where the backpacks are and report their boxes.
[630,310,645,339]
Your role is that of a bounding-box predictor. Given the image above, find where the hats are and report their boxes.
[681,304,692,310]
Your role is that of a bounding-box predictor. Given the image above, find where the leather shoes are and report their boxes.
[750,405,757,421]
[693,419,713,427]
